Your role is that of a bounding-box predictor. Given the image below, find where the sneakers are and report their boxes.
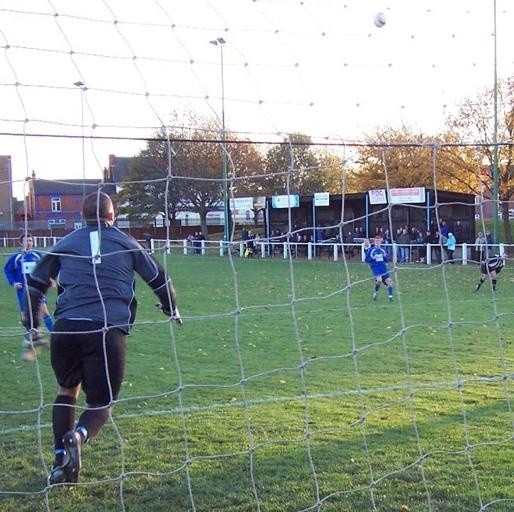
[45,466,62,484]
[62,430,84,483]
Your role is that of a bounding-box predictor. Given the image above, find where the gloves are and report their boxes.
[19,331,50,361]
[154,302,184,325]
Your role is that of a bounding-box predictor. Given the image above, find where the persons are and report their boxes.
[197,231,205,254]
[186,232,194,254]
[236,215,461,265]
[145,235,152,253]
[16,188,184,490]
[364,235,395,302]
[474,232,485,265]
[3,231,51,340]
[471,252,509,293]
[192,231,199,255]
[484,230,492,258]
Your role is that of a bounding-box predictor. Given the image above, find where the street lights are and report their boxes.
[73,80,88,226]
[208,36,235,252]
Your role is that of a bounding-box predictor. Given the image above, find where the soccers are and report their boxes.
[373,11,387,27]
